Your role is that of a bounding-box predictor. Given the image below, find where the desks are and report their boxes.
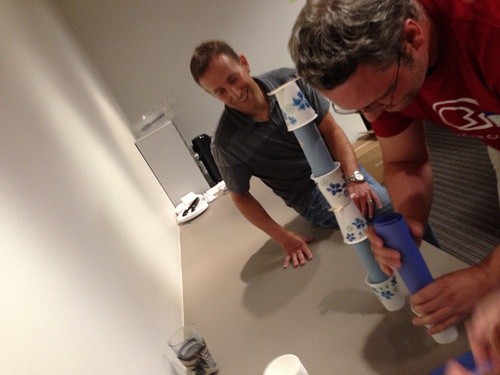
[179,172,477,375]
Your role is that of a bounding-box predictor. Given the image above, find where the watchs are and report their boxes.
[345,171,367,185]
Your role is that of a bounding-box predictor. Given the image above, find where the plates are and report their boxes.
[177,201,209,222]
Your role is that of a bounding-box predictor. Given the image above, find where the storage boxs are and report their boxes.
[351,133,385,186]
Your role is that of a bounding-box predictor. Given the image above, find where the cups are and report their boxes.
[275,81,318,132]
[263,354,308,375]
[331,203,369,245]
[310,162,351,210]
[168,324,219,375]
[365,268,405,311]
[411,303,458,344]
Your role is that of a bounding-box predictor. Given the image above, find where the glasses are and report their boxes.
[331,58,401,115]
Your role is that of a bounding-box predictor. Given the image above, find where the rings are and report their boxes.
[367,200,373,204]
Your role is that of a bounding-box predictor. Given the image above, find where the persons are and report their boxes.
[287,0,500,334]
[190,40,440,269]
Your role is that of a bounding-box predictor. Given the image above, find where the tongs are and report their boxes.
[182,197,199,216]
